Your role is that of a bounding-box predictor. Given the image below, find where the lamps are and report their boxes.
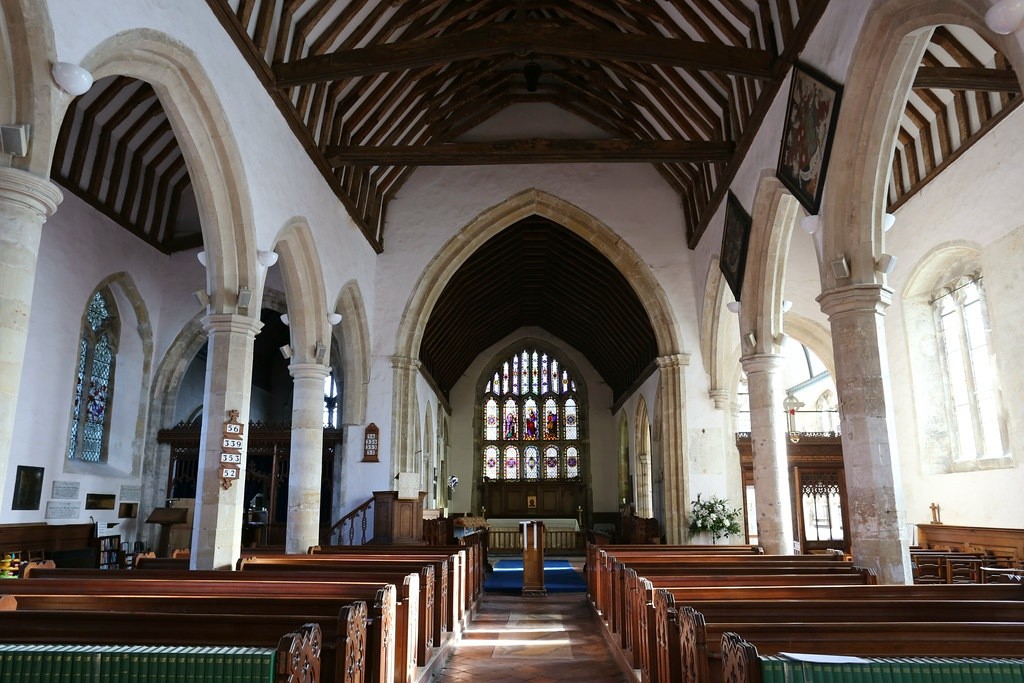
[884,212,895,231]
[783,300,793,314]
[197,250,343,326]
[51,61,94,97]
[727,301,742,313]
[800,214,819,233]
[984,0,1024,35]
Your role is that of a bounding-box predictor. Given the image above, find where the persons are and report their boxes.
[529,499,535,507]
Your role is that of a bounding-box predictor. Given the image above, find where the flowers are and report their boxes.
[688,493,743,540]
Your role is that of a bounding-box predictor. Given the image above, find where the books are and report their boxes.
[100,538,119,570]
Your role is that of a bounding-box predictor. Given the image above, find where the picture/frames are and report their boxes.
[719,187,753,300]
[775,59,845,216]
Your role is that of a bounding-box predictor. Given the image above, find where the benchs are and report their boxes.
[584,540,1024,683]
[0,539,485,683]
